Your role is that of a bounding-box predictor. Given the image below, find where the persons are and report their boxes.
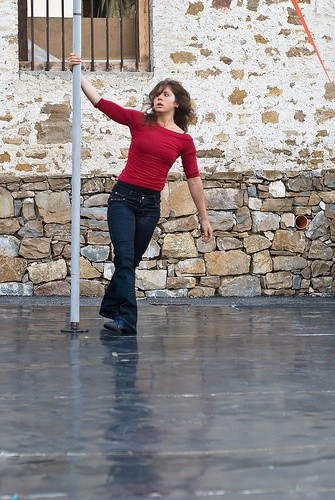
[67,51,214,336]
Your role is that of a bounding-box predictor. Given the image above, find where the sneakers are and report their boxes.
[103,319,136,334]
